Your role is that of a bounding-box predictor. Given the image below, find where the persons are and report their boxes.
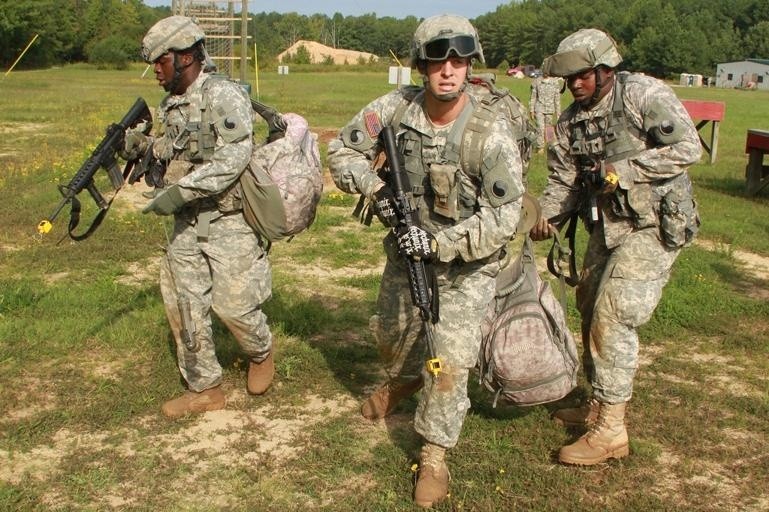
[116,12,276,420]
[526,27,703,464]
[326,14,526,508]
[528,72,561,153]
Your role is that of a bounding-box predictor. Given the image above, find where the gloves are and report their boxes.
[392,225,439,264]
[118,129,147,161]
[370,185,409,230]
[141,183,186,218]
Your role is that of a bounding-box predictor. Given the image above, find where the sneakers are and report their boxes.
[556,397,631,467]
[412,441,452,506]
[360,371,426,421]
[160,384,226,419]
[553,394,607,426]
[247,340,275,396]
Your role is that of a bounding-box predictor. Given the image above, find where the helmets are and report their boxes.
[140,14,207,63]
[409,14,487,70]
[542,28,623,79]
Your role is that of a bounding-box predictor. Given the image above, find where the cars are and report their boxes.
[528,70,543,78]
[506,65,524,75]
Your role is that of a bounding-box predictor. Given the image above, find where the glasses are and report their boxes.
[417,34,480,62]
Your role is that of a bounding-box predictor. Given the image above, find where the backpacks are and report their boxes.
[395,72,537,190]
[205,79,325,240]
[481,225,581,409]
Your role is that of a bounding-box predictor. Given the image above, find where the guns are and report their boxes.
[37,98,153,236]
[378,125,442,376]
[578,152,606,224]
